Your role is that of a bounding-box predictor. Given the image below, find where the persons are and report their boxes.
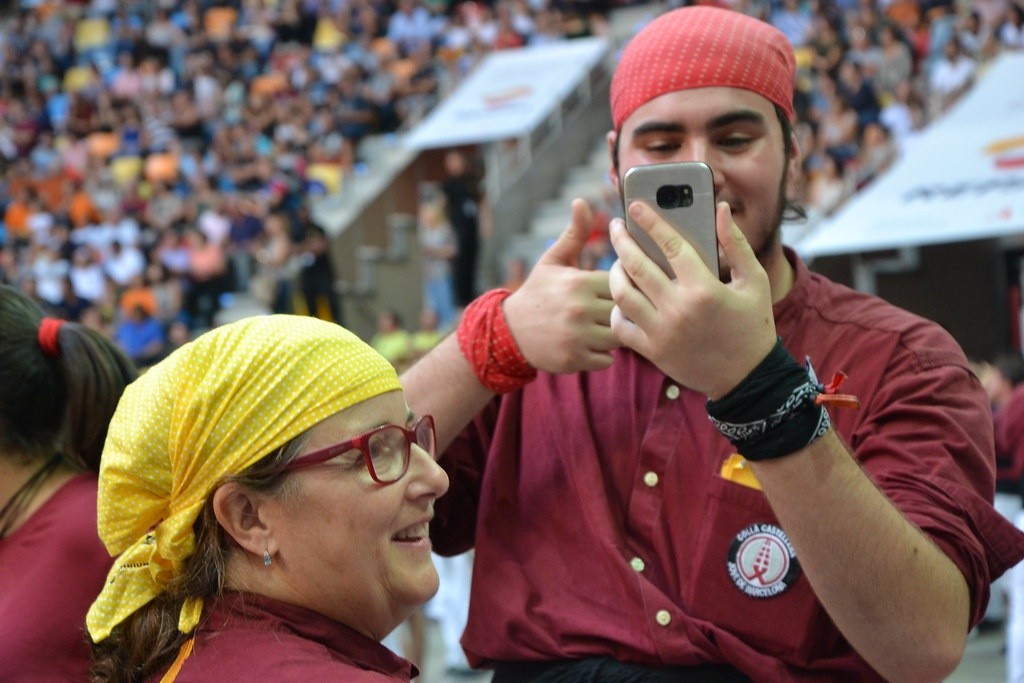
[0,1,1022,683]
[84,313,449,683]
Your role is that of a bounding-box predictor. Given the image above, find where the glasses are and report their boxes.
[281,414,436,485]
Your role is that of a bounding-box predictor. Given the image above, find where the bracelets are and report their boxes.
[704,340,830,461]
[457,287,537,394]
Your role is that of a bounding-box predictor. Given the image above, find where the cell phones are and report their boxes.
[624,161,721,282]
